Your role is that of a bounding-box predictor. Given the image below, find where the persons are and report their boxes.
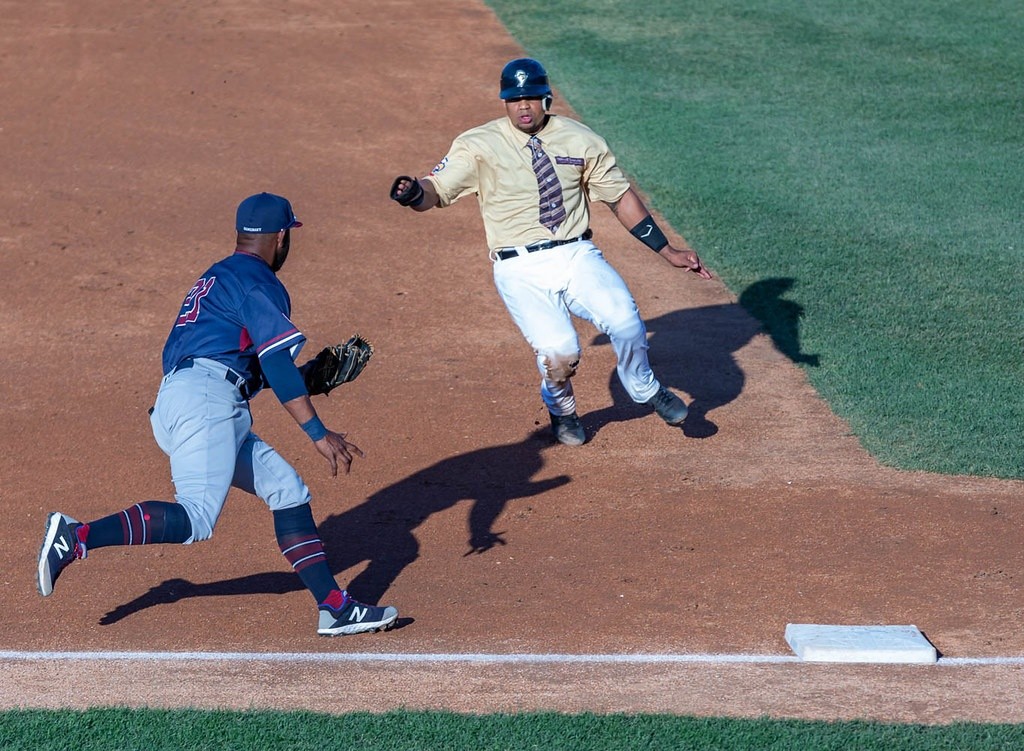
[389,58,714,446]
[37,189,399,634]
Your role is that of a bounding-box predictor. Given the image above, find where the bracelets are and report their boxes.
[299,413,329,441]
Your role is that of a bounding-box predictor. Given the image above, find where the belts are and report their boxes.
[169,358,249,401]
[494,229,593,260]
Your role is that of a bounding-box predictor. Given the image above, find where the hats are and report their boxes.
[500,58,551,99]
[236,191,303,234]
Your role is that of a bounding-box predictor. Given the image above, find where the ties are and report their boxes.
[526,137,566,235]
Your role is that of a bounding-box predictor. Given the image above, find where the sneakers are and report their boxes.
[318,591,398,637]
[548,411,587,444]
[647,386,688,423]
[37,511,87,597]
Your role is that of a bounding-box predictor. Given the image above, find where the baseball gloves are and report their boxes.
[297,331,373,399]
